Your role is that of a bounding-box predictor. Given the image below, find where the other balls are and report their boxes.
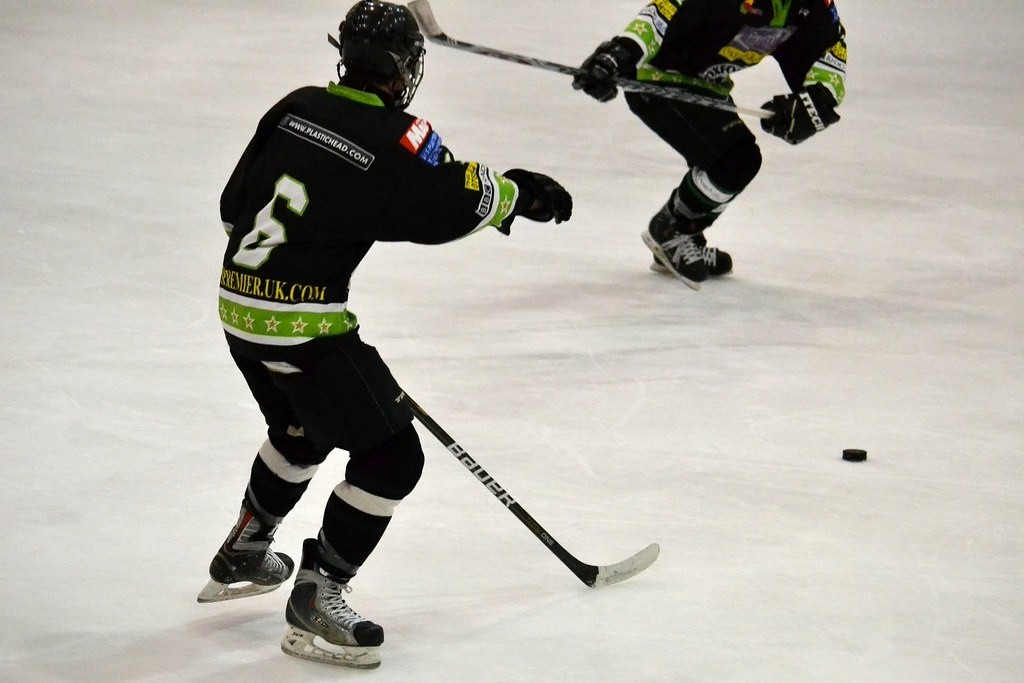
[842,449,867,463]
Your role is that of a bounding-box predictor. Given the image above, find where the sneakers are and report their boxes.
[279,538,384,669]
[196,485,296,603]
[649,231,731,275]
[641,191,707,290]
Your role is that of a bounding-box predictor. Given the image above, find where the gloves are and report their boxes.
[759,84,839,146]
[572,40,636,104]
[500,167,574,224]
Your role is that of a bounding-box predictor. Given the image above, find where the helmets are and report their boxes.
[338,1,426,108]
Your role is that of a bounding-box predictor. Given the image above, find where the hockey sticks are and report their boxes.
[394,380,664,594]
[405,0,783,136]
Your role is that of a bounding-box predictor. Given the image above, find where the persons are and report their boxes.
[573,0,847,291]
[197,0,571,670]
